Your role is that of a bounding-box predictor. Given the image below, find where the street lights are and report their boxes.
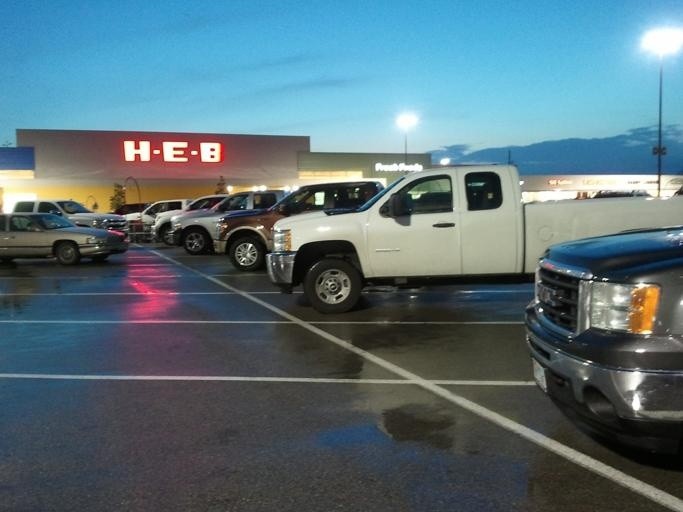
[639,23,681,200]
[395,110,418,160]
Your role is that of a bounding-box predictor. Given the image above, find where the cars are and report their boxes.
[0,199,130,265]
[524,225,683,455]
